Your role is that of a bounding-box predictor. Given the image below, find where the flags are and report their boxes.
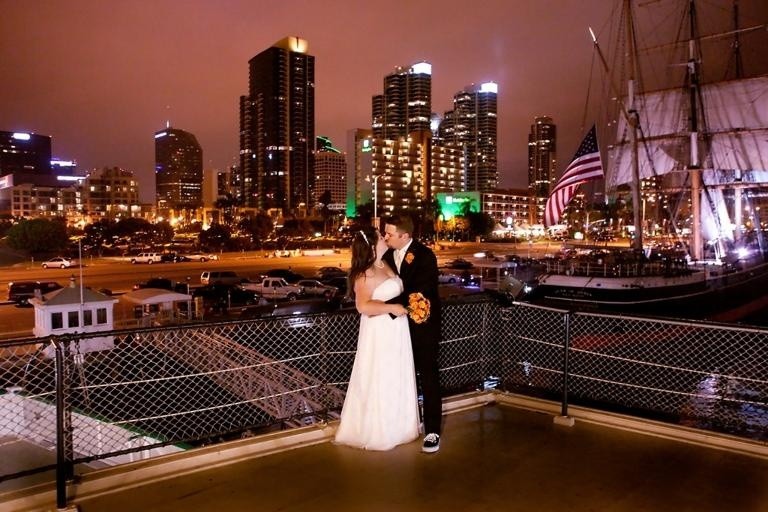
[543,124,602,225]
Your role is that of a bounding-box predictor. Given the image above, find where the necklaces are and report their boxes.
[374,261,385,269]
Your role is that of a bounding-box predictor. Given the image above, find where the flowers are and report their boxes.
[404,252,415,264]
[404,292,432,325]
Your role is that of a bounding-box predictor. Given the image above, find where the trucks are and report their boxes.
[5,279,63,308]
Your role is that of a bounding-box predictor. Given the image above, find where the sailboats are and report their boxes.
[531,1,766,318]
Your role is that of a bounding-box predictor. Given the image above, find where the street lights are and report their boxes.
[373,171,386,230]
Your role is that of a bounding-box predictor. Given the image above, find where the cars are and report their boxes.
[132,266,352,308]
[435,229,687,294]
[40,256,76,269]
[123,251,220,263]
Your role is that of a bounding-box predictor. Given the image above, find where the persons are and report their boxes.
[332,226,424,453]
[366,214,443,453]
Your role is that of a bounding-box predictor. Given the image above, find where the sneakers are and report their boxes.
[422,433,440,453]
